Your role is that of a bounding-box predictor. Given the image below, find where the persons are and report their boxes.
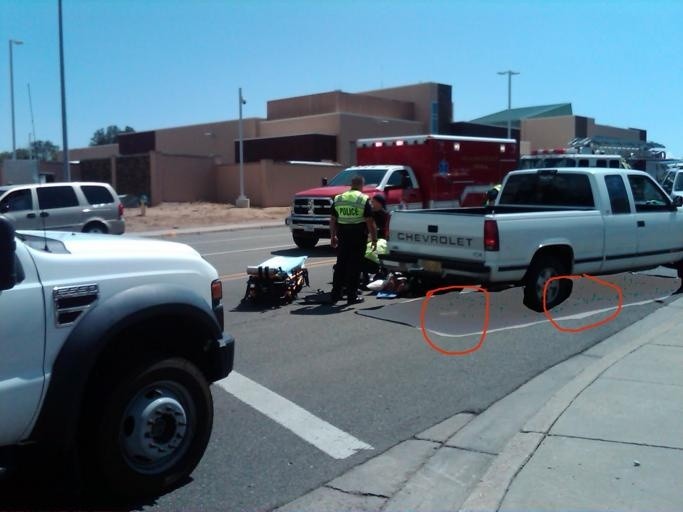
[321,175,376,307]
[358,193,387,291]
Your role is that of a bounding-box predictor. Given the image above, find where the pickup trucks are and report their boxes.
[382,135,683,316]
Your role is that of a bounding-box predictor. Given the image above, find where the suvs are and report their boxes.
[0,213,238,491]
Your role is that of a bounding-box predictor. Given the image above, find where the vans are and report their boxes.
[1,180,128,237]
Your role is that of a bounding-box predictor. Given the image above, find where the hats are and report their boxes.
[374,195,386,208]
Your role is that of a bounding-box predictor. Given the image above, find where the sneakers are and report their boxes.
[348,297,364,304]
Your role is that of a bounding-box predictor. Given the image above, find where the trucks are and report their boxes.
[281,131,523,255]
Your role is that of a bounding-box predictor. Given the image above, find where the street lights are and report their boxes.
[496,70,521,140]
[234,86,251,208]
[8,38,24,160]
